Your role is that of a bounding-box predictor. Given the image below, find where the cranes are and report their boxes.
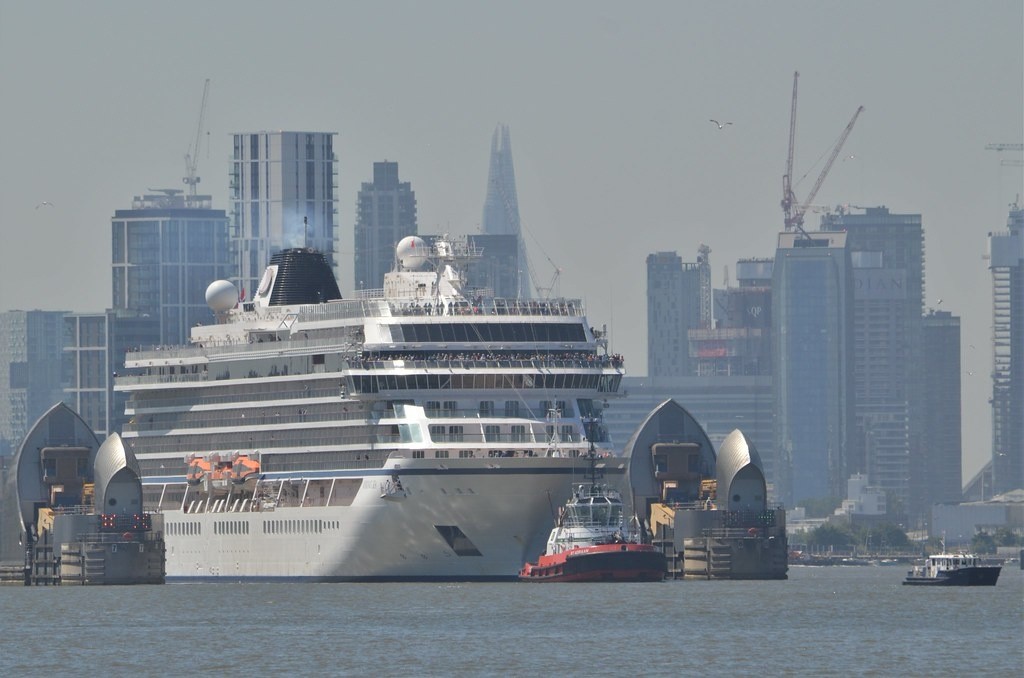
[182,78,213,208]
[782,72,801,231]
[793,106,865,228]
[984,141,1024,213]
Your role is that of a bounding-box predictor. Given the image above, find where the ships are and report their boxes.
[113,215,629,582]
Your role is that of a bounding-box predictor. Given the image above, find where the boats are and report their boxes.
[517,420,668,582]
[7,401,166,585]
[802,557,898,566]
[902,553,1001,586]
[624,398,790,581]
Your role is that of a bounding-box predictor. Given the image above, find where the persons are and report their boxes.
[410,295,572,315]
[351,353,624,360]
